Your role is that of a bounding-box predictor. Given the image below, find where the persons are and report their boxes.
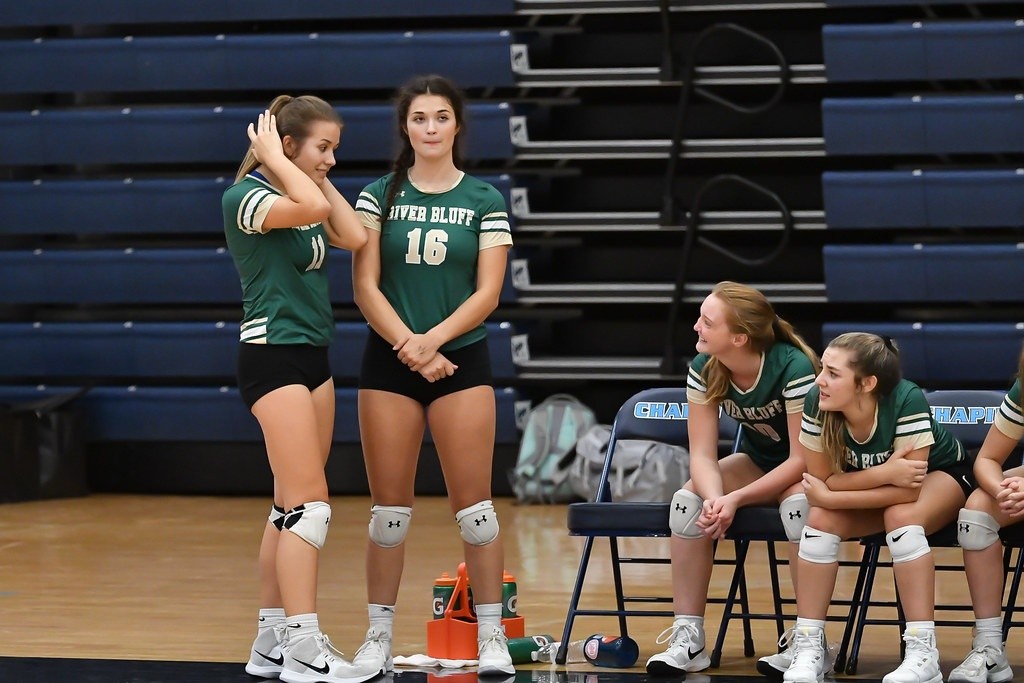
[646,282,829,680]
[949,347,1024,683]
[219,96,383,683]
[782,332,973,683]
[352,75,516,677]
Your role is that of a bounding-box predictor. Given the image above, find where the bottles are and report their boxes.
[502,569,517,618]
[531,639,587,663]
[583,634,639,668]
[506,635,556,665]
[431,573,457,619]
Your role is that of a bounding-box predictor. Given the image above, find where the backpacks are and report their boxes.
[510,392,597,504]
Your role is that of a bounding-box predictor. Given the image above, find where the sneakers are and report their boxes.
[882,629,944,683]
[245,627,292,679]
[476,623,515,675]
[278,630,383,683]
[783,623,825,683]
[646,618,712,682]
[948,641,1014,683]
[351,623,394,672]
[755,621,833,677]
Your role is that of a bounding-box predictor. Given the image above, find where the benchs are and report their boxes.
[0,323,513,382]
[0,29,514,89]
[823,96,1024,154]
[824,19,1024,83]
[0,250,513,307]
[0,103,516,167]
[0,175,513,237]
[824,323,1024,384]
[822,168,1024,229]
[0,387,518,499]
[823,243,1024,303]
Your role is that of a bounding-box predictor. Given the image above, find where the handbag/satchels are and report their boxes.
[568,424,691,502]
[0,390,88,502]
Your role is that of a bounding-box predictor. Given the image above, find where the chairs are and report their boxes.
[705,389,924,673]
[846,390,1024,676]
[555,388,756,668]
